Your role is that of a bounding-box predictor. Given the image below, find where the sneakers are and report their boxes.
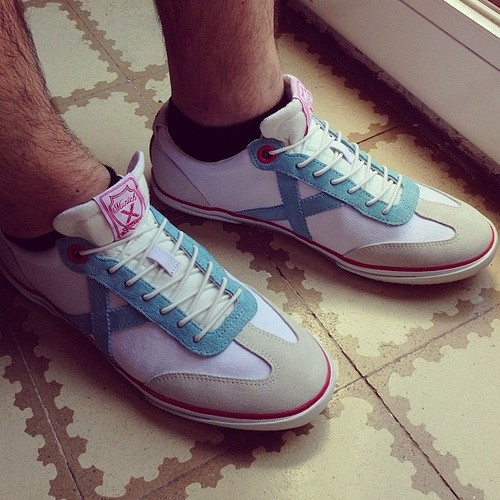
[149,75,499,285]
[0,150,333,431]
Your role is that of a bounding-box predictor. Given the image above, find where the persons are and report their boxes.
[1,0,499,433]
